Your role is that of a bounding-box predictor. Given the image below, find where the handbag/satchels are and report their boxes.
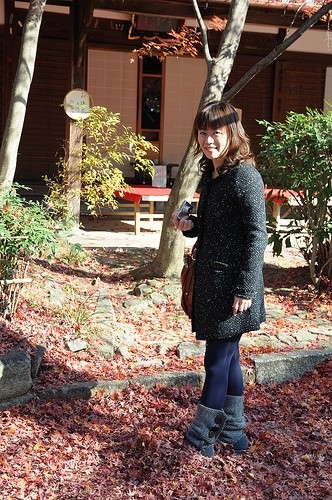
[181,253,195,319]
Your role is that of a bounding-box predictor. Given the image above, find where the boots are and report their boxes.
[182,403,227,457]
[219,395,248,451]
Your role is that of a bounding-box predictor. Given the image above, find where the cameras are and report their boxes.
[175,200,193,221]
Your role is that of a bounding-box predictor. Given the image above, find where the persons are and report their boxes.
[172,100,268,457]
